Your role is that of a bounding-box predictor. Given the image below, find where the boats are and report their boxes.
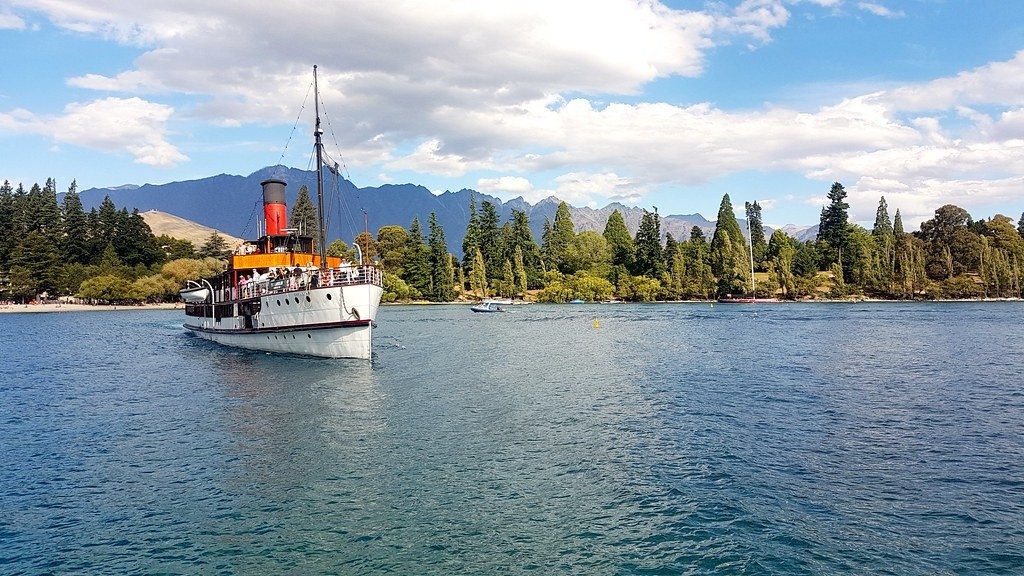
[569,299,584,304]
[483,297,513,305]
[180,286,208,303]
[471,300,506,313]
[599,297,625,304]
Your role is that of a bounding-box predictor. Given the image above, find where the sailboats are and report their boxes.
[718,216,756,303]
[179,65,383,361]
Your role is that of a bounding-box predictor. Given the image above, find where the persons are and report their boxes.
[237,258,382,298]
[114,298,144,306]
[0,298,47,306]
[238,242,294,255]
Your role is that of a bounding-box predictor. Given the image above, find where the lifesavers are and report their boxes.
[321,271,330,283]
[352,308,360,320]
